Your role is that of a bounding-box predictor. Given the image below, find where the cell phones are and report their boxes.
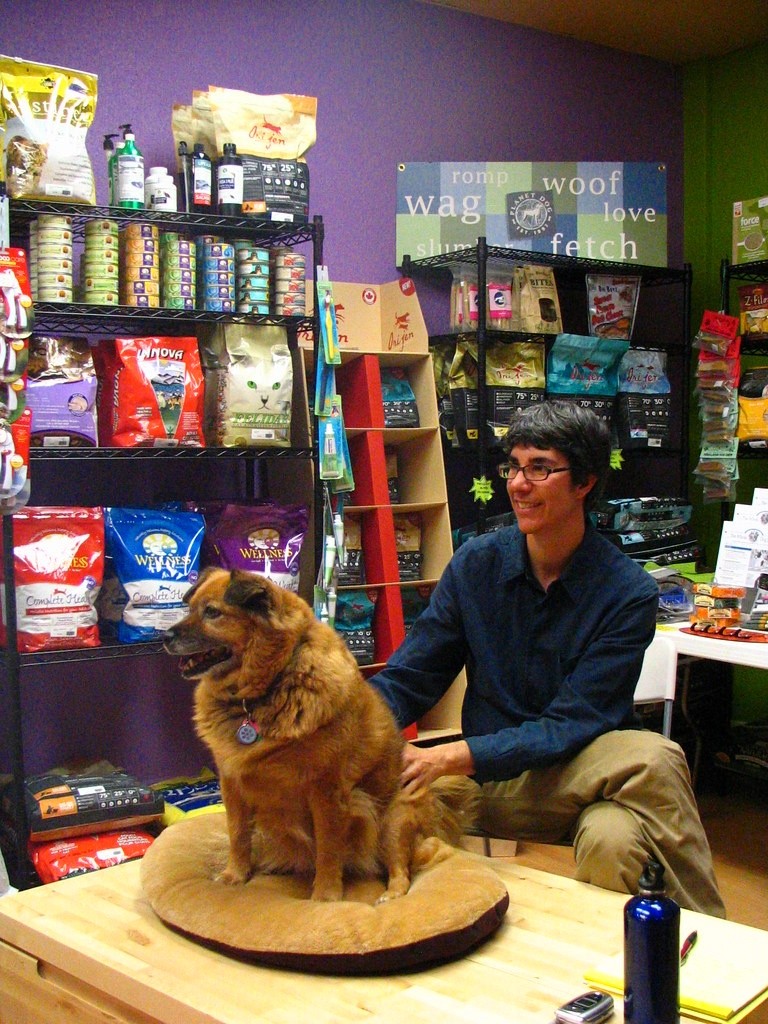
[555,990,615,1024]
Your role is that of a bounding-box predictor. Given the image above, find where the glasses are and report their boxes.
[495,462,573,481]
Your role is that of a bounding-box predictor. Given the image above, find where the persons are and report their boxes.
[371,401,726,919]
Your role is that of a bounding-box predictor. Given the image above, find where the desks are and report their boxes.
[655,620,768,738]
[0,844,767,1023]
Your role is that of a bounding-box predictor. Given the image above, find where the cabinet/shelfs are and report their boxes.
[1,180,767,891]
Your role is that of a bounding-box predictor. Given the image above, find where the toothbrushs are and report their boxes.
[309,258,352,630]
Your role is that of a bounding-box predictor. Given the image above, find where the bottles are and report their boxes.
[623,858,680,1024]
[99,124,244,217]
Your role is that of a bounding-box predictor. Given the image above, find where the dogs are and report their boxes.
[160,564,483,905]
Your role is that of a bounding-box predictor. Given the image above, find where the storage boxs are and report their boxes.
[28,214,306,317]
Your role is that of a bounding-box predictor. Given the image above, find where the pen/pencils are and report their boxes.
[681,929,698,967]
